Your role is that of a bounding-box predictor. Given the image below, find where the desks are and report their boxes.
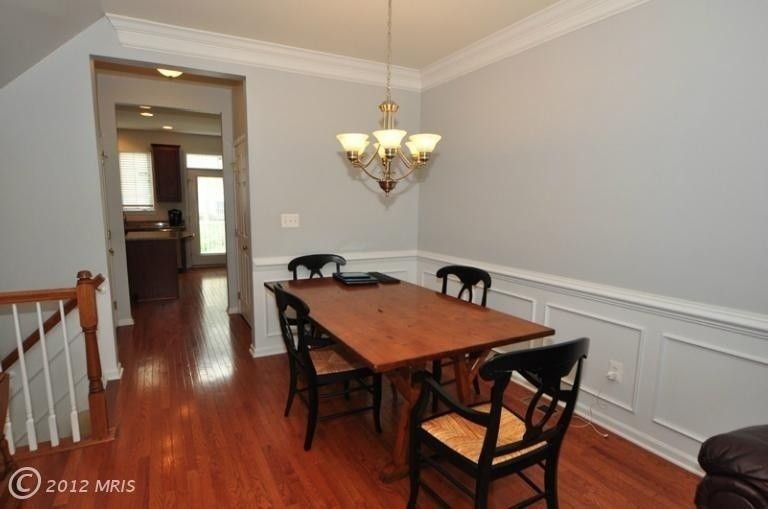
[264,271,557,484]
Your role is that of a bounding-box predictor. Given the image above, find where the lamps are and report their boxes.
[336,0,443,199]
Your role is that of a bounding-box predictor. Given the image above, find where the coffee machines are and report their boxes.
[167,209,182,226]
